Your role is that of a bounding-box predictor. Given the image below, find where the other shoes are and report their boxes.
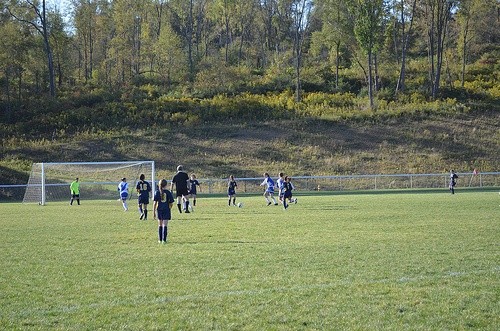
[140,213,145,220]
[274,203,278,205]
[267,202,272,206]
[158,241,166,244]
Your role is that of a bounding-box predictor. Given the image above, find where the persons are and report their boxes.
[260,173,278,205]
[118,178,129,212]
[153,179,174,244]
[227,175,238,206]
[449,169,458,195]
[276,173,297,209]
[182,174,200,212]
[136,174,150,220]
[171,165,190,213]
[70,178,80,205]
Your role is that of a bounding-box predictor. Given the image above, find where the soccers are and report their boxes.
[237,202,244,208]
